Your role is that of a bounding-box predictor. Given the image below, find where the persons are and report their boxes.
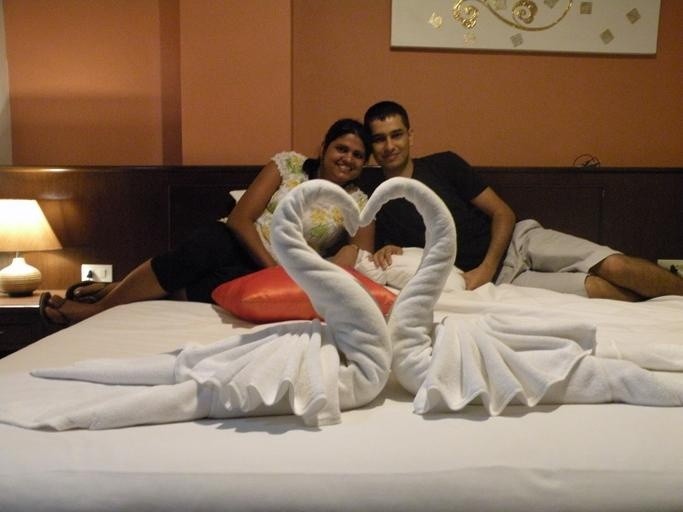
[36,115,371,332]
[359,97,682,306]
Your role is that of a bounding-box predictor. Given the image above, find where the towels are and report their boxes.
[360,175,682,429]
[1,172,391,430]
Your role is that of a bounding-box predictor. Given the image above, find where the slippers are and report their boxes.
[65,277,122,305]
[38,290,71,332]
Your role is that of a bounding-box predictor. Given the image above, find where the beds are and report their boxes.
[0,259,682,512]
